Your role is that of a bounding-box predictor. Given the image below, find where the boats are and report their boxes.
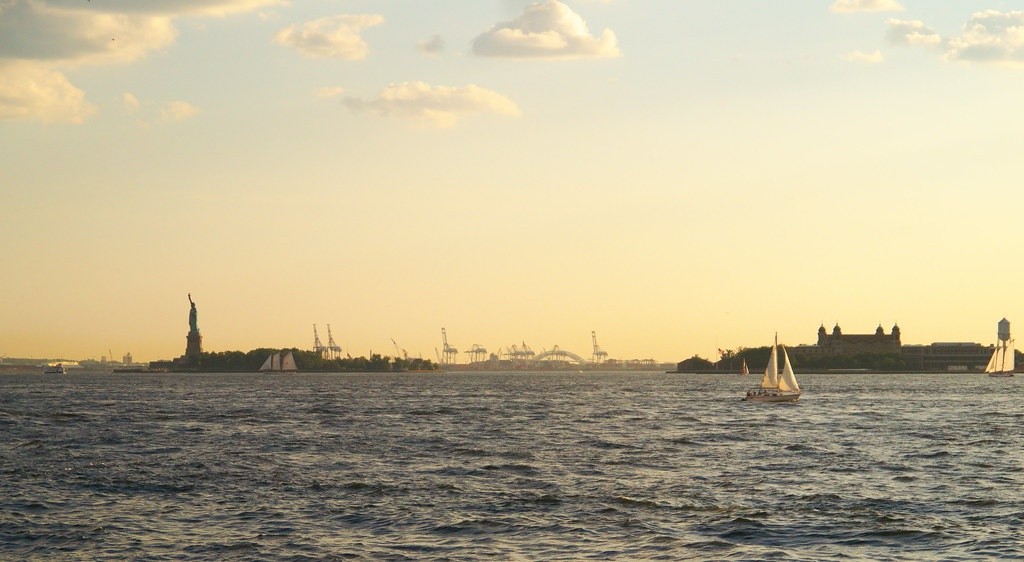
[44,364,67,375]
[113,363,148,373]
[47,360,79,369]
[578,370,583,374]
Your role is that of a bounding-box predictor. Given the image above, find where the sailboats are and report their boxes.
[258,351,298,376]
[740,331,801,403]
[984,339,1015,378]
[737,357,749,375]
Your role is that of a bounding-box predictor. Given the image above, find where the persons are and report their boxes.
[188,292,197,331]
